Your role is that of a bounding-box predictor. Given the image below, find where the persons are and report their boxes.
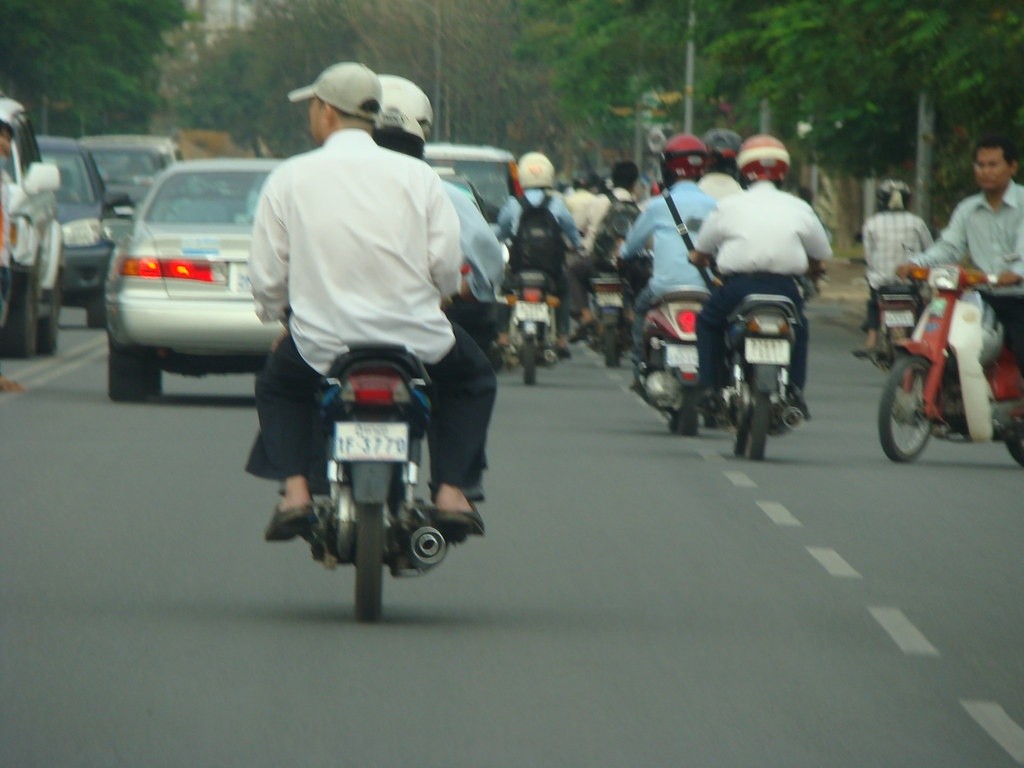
[852,180,934,357]
[689,134,833,422]
[0,119,25,391]
[897,136,1024,377]
[244,74,506,505]
[254,61,497,543]
[495,127,745,390]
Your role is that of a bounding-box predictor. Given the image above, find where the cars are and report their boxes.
[108,159,289,404]
[1,92,178,358]
[423,147,528,218]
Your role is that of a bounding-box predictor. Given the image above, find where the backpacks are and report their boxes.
[508,193,566,276]
[594,189,644,272]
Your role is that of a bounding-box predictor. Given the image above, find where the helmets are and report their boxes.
[735,133,790,181]
[706,128,742,164]
[978,302,1006,362]
[663,135,708,158]
[375,72,435,128]
[878,178,911,210]
[518,152,554,189]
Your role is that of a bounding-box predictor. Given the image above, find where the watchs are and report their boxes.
[987,273,999,290]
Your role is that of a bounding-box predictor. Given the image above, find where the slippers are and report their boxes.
[265,506,310,542]
[431,497,485,535]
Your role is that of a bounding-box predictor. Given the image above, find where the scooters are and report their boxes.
[502,230,824,465]
[848,253,1023,468]
[277,305,466,621]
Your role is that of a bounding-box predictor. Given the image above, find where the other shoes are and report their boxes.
[493,343,508,355]
[556,343,572,360]
[852,345,876,358]
[791,383,812,420]
[684,376,721,393]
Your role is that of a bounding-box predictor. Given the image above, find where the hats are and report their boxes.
[288,61,382,119]
